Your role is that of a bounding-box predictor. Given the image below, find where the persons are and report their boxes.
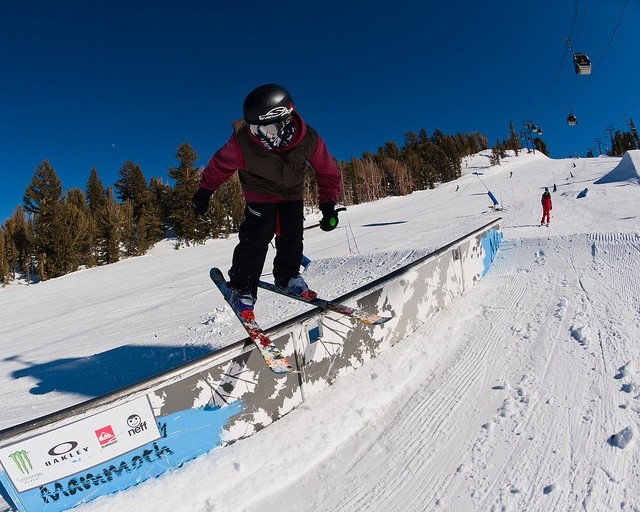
[191,83,341,310]
[541,187,552,225]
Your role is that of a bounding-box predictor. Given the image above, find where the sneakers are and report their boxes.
[275,273,308,296]
[226,278,253,315]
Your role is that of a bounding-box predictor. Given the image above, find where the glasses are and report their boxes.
[283,116,292,124]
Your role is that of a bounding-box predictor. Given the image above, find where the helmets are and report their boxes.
[243,83,295,125]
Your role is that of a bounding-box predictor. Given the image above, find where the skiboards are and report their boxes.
[210,267,393,374]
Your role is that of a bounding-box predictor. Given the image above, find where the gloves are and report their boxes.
[319,207,347,231]
[192,188,213,214]
